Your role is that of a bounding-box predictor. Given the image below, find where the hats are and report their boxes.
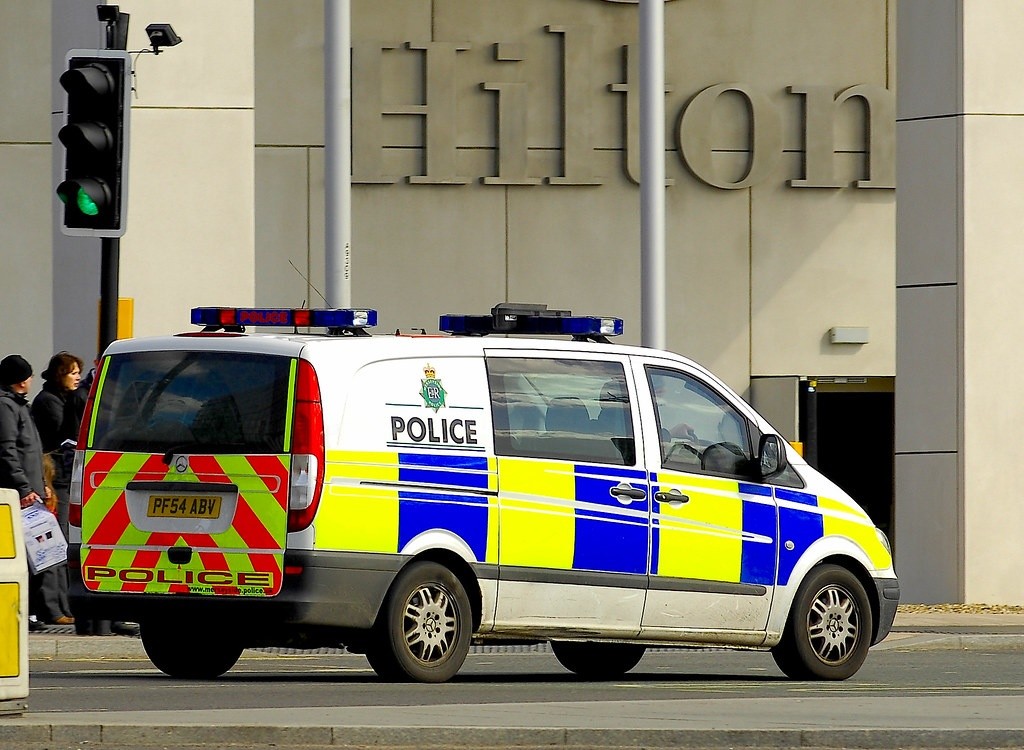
[0,355,32,385]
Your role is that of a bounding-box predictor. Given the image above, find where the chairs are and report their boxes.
[534,396,626,466]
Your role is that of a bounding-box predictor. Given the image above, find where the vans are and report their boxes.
[67,304,900,682]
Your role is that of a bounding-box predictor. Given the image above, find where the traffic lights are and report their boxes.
[57,49,131,238]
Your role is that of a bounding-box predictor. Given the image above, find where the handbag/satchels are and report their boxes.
[21,498,68,576]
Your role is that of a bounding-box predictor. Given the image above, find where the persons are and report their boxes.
[30,350,105,625]
[598,380,694,465]
[110,355,263,453]
[0,354,51,631]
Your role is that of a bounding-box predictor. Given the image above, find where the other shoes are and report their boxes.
[29,619,45,630]
[47,616,75,624]
